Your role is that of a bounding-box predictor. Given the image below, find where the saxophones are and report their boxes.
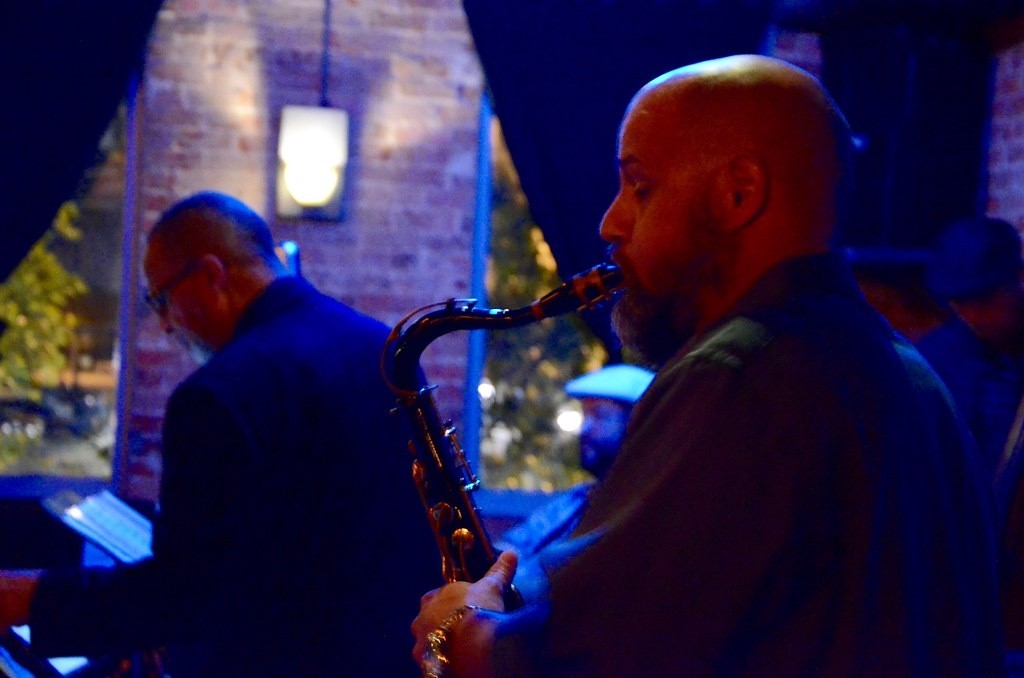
[381,263,624,612]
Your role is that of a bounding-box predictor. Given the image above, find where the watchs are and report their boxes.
[418,601,501,678]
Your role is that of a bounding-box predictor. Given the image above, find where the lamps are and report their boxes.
[276,104,347,223]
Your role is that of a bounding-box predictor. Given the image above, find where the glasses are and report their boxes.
[143,260,204,312]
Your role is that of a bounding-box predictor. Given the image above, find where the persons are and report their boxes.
[475,364,666,627]
[410,55,1010,678]
[812,220,1021,628]
[914,178,1024,586]
[0,190,464,678]
